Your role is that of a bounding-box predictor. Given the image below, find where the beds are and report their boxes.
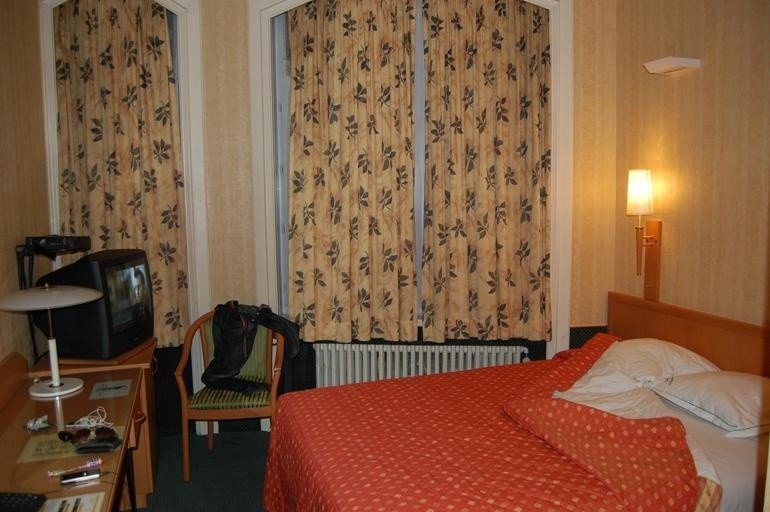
[262,289,770,512]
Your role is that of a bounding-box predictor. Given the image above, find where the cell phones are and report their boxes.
[60,468,101,484]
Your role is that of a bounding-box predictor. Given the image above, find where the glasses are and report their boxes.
[57,428,92,443]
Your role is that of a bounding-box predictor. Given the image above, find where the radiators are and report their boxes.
[311,343,530,388]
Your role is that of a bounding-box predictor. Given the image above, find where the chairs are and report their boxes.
[174,309,285,482]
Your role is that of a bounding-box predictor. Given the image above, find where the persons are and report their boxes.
[131,269,151,320]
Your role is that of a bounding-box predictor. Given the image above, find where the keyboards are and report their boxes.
[0,492,48,512]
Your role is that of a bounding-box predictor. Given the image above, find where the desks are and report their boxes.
[0,351,145,512]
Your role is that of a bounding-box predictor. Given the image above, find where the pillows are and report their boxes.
[620,337,721,385]
[645,370,770,439]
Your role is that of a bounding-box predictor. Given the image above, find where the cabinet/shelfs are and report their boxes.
[29,336,159,512]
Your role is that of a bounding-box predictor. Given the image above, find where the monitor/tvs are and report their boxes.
[33,249,154,360]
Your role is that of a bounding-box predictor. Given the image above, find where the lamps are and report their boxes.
[625,168,662,303]
[0,282,104,398]
